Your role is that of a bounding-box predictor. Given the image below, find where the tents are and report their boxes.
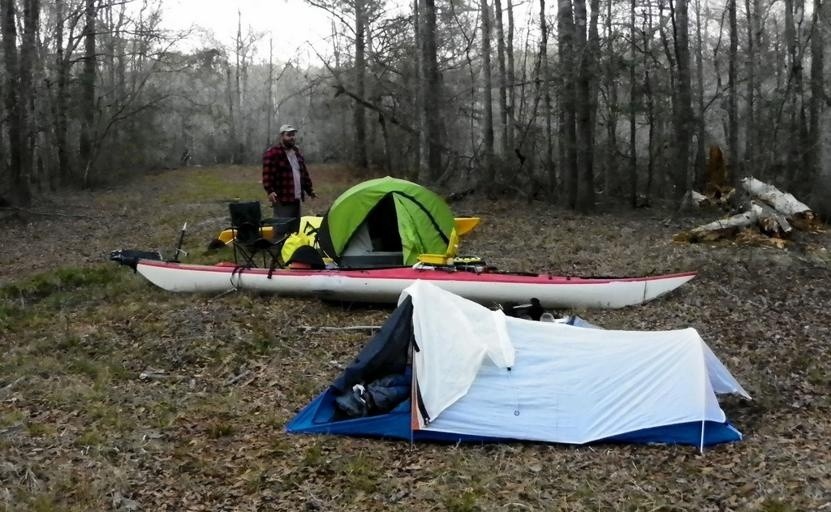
[286,278,752,448]
[320,175,458,266]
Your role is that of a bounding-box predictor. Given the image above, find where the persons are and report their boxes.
[706,142,724,206]
[263,125,319,269]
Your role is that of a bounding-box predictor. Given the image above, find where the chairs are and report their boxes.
[229,202,297,269]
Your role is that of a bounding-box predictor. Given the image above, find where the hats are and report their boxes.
[279,124,297,134]
